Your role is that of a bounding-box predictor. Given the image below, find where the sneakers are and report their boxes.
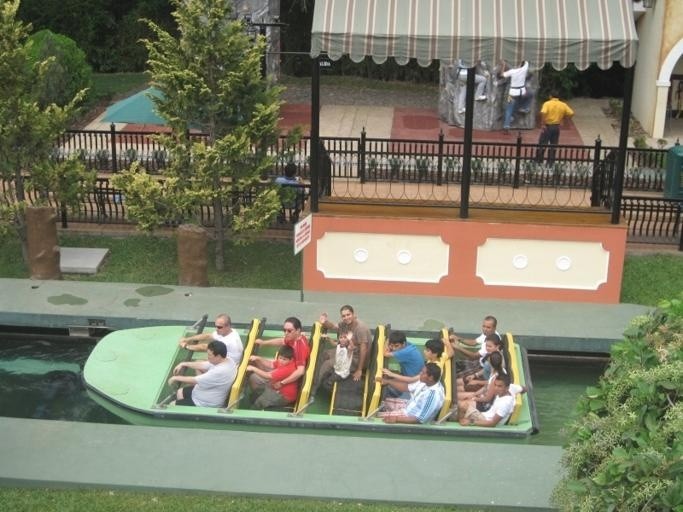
[474,96,487,100]
[459,108,465,113]
[518,108,529,113]
[503,128,509,134]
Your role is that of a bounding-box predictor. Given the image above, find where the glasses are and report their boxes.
[215,326,223,329]
[284,328,293,333]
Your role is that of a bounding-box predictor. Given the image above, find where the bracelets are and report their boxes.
[184,343,189,350]
[280,380,287,385]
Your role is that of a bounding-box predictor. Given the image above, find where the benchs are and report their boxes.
[69,185,301,220]
[229,319,524,426]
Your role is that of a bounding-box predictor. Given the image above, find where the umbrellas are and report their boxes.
[101,86,206,132]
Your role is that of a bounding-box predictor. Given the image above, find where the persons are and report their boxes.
[245,344,298,410]
[179,313,244,375]
[321,329,357,403]
[530,88,574,169]
[246,316,310,392]
[498,60,531,136]
[371,315,528,429]
[308,304,371,403]
[166,340,238,407]
[273,163,306,225]
[456,59,489,114]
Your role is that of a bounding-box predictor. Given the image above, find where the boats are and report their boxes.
[82,314,540,444]
